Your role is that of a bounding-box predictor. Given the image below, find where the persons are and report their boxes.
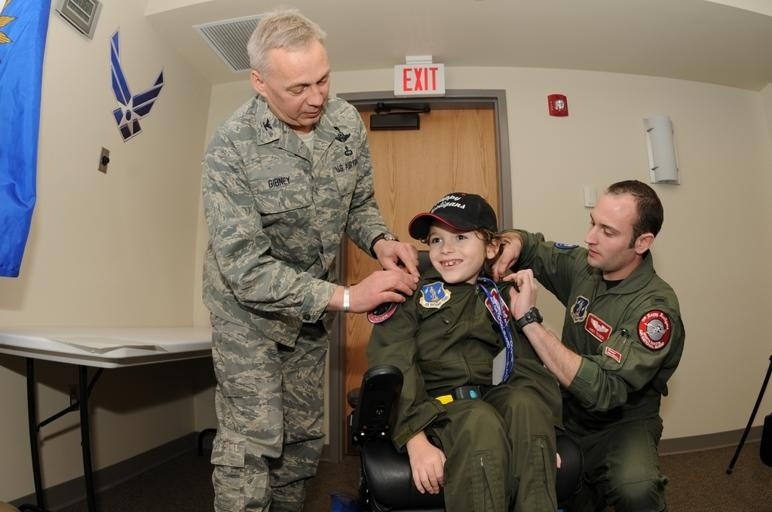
[483,178,687,511]
[360,193,562,511]
[196,5,420,511]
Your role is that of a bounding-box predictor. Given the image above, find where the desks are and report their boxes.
[0,322,211,511]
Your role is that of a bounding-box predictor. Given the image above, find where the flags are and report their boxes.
[0,0,46,282]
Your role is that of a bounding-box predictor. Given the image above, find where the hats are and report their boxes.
[409,192,498,240]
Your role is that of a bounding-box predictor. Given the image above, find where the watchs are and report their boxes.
[515,305,544,333]
[370,231,400,260]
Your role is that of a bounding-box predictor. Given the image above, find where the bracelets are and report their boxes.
[342,281,355,317]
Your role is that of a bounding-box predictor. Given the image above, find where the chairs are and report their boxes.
[346,250,587,512]
